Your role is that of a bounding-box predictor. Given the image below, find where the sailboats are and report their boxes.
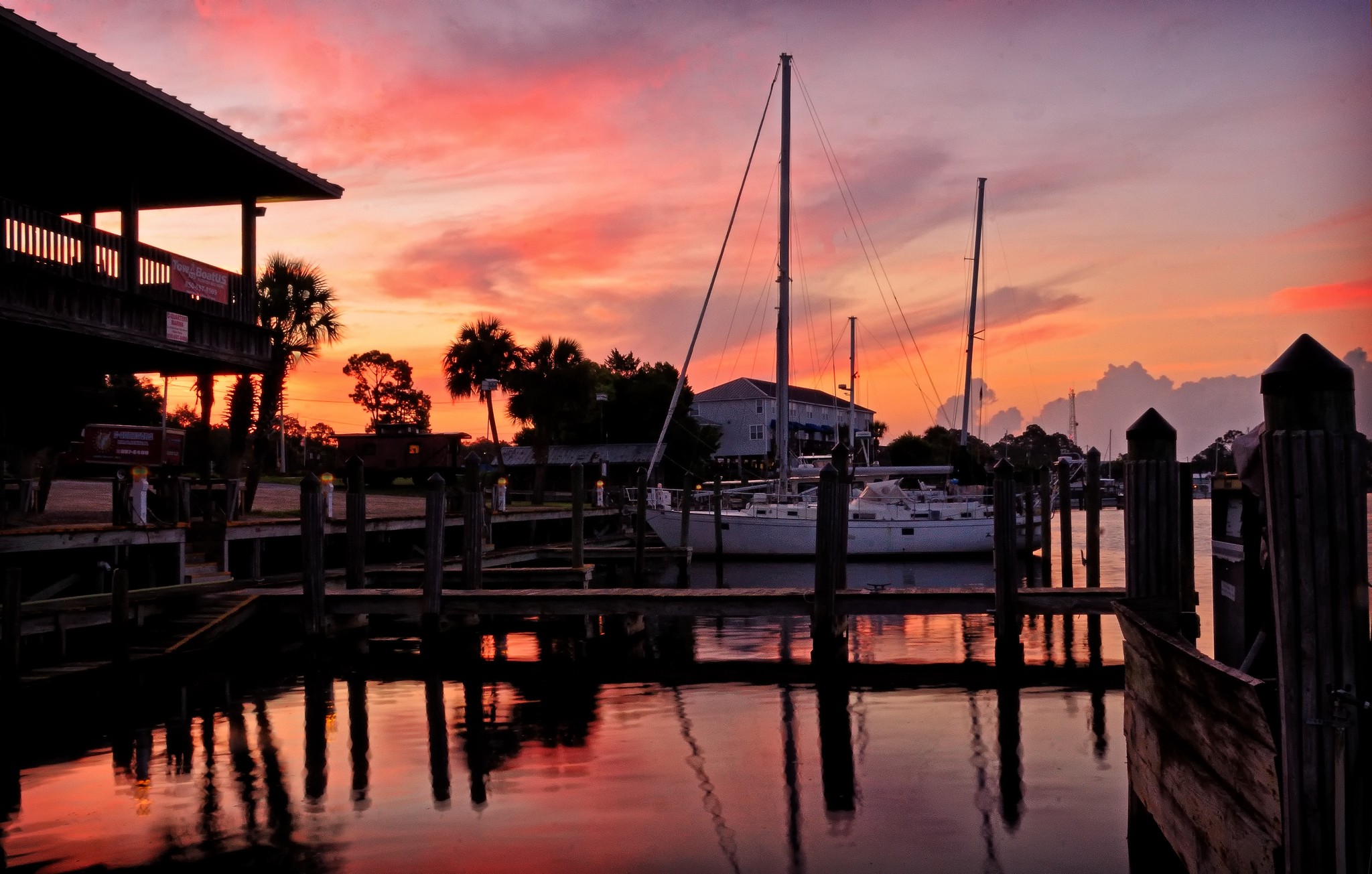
[619,51,1051,558]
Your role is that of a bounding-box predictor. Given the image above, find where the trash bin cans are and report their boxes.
[1211,424,1372,669]
[447,475,465,515]
